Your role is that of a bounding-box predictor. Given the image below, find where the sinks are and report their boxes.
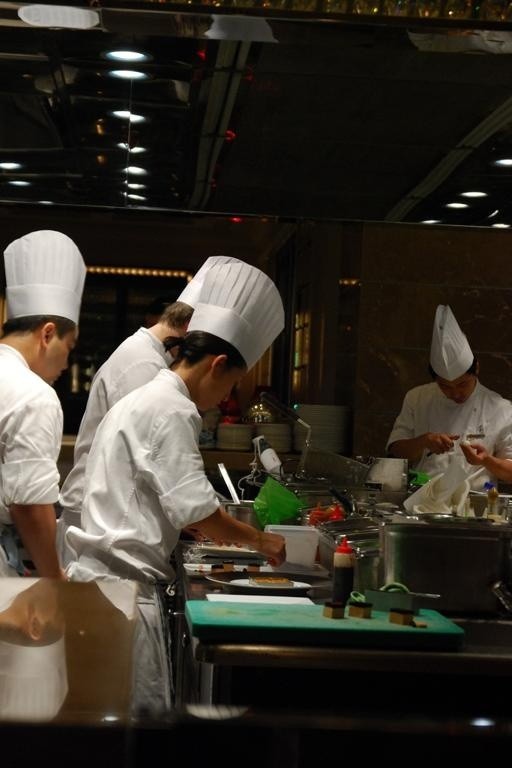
[207,472,262,505]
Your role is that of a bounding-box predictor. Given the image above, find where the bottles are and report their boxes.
[484,481,500,521]
[332,536,357,604]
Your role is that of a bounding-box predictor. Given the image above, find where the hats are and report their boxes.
[185,263,286,375]
[4,229,88,327]
[176,255,249,312]
[429,303,475,383]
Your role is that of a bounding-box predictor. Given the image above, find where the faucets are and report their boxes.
[261,390,312,480]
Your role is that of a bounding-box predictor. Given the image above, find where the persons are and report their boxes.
[376,351,511,496]
[0,313,83,578]
[49,301,196,531]
[63,332,290,722]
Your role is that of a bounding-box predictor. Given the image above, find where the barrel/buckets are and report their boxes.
[357,456,408,501]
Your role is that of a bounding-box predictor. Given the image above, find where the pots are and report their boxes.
[220,498,264,533]
[202,569,335,594]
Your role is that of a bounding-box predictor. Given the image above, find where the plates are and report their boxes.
[214,402,347,453]
[230,577,312,588]
